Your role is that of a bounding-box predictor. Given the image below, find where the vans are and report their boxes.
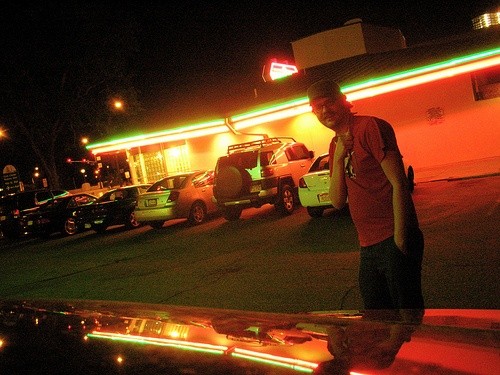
[0,189,69,240]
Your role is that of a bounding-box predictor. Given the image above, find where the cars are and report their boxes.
[135,171,218,228]
[298,153,348,218]
[26,194,99,236]
[78,184,153,233]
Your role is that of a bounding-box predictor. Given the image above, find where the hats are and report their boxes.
[307,80,341,105]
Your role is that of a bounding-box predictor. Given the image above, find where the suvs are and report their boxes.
[213,137,316,220]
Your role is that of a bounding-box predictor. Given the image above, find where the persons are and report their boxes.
[307,79,423,308]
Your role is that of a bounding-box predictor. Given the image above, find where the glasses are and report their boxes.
[312,97,336,114]
